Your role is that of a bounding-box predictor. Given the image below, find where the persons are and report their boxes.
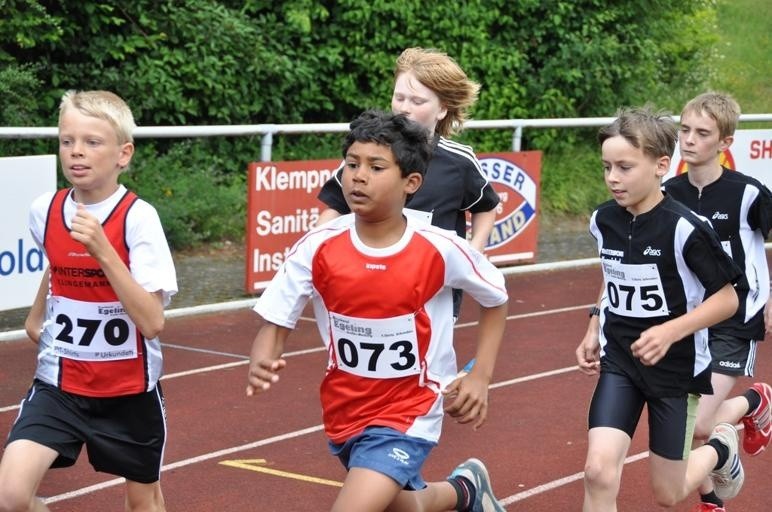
[576,107,749,512]
[245,109,510,512]
[315,48,502,331]
[0,84,182,512]
[660,91,772,512]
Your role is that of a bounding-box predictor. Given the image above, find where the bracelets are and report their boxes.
[589,306,600,317]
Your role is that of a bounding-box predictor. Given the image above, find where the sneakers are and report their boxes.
[743,383,772,457]
[689,501,726,512]
[708,422,745,500]
[447,458,506,512]
